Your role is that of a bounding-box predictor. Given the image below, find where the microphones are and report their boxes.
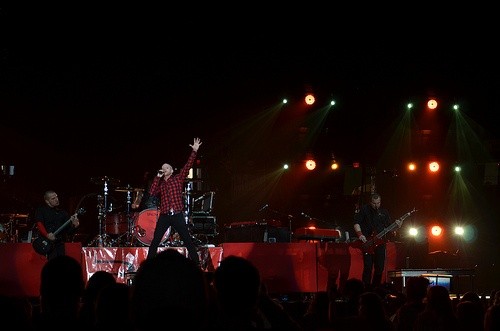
[259,204,269,211]
[301,212,312,220]
[157,171,164,174]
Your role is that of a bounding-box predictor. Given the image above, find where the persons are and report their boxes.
[352,193,404,291]
[36,190,80,262]
[146,138,203,264]
[0,248,499,331]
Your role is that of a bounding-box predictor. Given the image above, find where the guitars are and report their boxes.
[349,205,421,256]
[33,205,89,255]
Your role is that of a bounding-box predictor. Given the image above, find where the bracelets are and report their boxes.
[356,231,363,237]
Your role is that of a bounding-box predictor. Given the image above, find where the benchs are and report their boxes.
[292,228,339,244]
[224,220,282,243]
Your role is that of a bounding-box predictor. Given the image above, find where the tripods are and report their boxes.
[88,181,144,248]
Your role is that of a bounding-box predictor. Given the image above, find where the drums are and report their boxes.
[129,208,172,247]
[101,206,131,238]
[185,180,209,194]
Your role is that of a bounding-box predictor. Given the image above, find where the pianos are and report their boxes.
[388,266,478,301]
[292,228,341,243]
